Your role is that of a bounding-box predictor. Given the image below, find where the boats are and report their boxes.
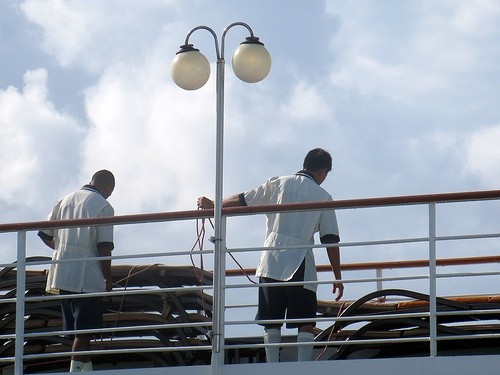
[0,257,216,371]
[314,289,500,357]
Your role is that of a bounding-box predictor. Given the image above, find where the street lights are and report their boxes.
[170,21,272,365]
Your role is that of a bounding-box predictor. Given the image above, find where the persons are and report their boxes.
[197,148,345,363]
[36,169,115,372]
